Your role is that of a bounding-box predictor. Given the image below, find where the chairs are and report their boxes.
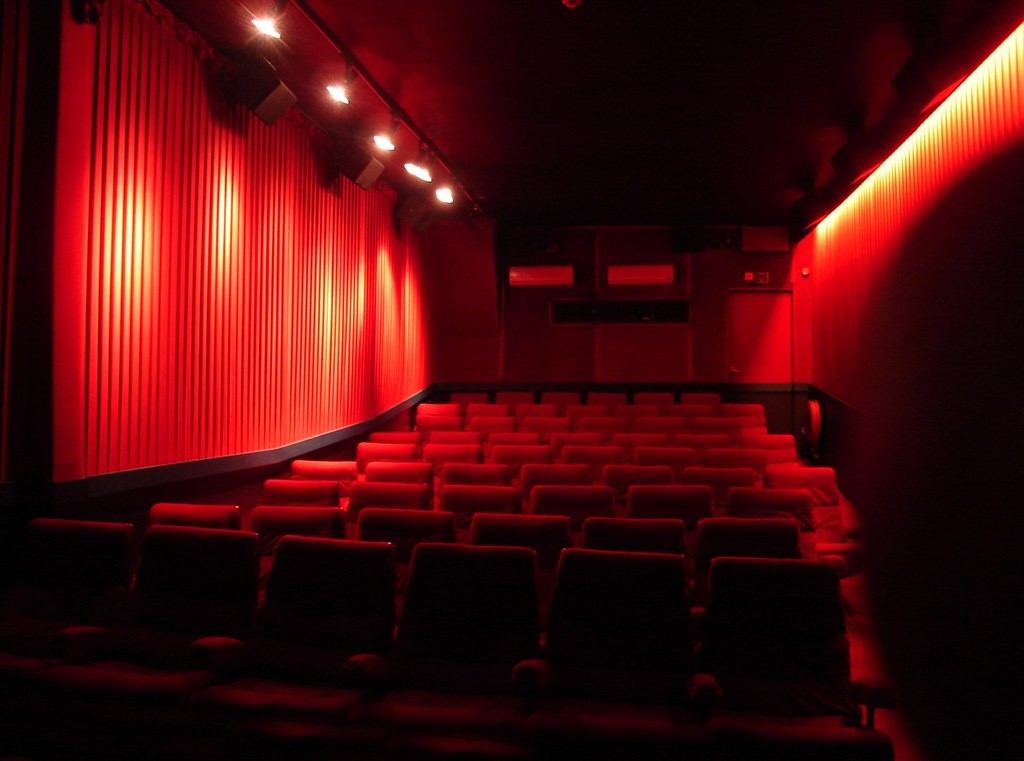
[0,391,900,761]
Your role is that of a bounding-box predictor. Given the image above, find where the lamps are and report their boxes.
[404,138,438,184]
[326,69,357,105]
[251,0,290,38]
[374,120,400,151]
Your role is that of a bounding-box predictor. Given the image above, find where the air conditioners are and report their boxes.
[508,265,575,288]
[607,264,676,286]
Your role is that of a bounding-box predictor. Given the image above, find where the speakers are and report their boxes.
[342,144,384,191]
[234,60,297,126]
[395,195,421,221]
